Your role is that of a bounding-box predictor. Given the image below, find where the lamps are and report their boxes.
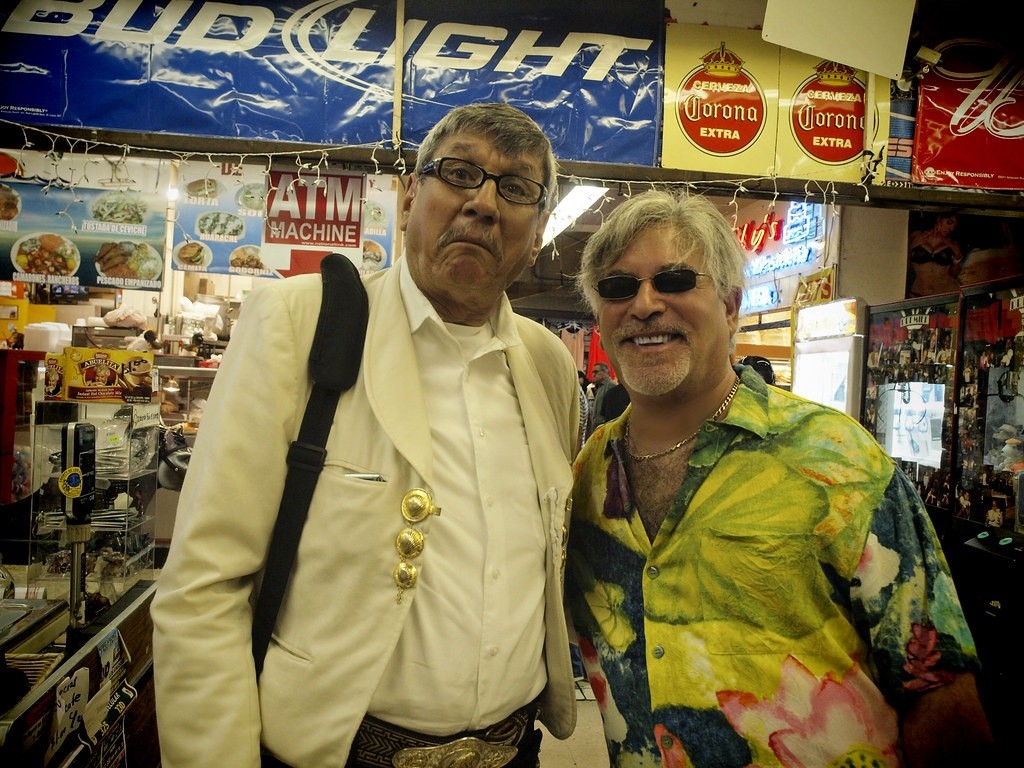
[538,183,612,250]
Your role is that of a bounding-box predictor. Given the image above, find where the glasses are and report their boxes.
[592,270,713,301]
[418,157,548,206]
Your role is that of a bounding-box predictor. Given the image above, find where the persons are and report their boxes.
[576,352,777,453]
[866,319,1023,533]
[563,191,996,768]
[148,101,583,768]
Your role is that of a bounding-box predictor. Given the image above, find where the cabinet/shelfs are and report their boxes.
[0,346,165,768]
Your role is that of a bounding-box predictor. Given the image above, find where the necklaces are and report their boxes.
[622,371,741,463]
